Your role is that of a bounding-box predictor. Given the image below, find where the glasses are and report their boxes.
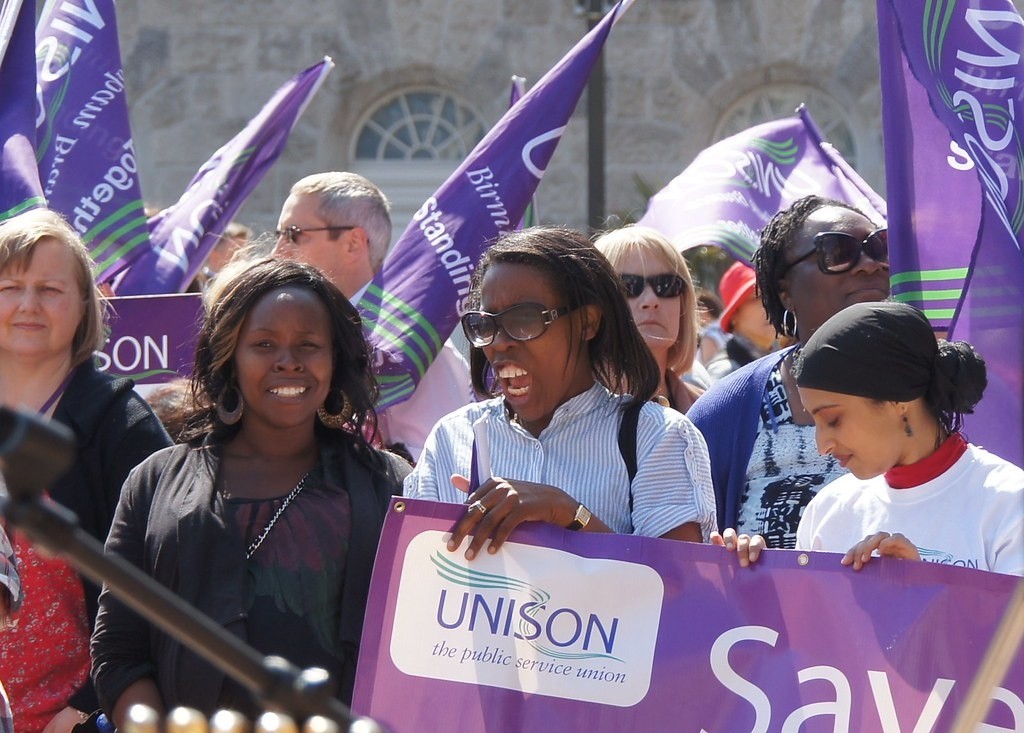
[275,224,370,244]
[785,228,888,275]
[461,301,571,347]
[616,273,685,299]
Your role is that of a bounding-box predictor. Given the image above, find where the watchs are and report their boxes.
[565,502,594,531]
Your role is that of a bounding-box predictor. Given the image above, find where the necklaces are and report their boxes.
[243,470,311,560]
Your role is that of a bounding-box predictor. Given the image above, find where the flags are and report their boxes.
[632,104,888,263]
[1,0,620,413]
[856,5,1022,467]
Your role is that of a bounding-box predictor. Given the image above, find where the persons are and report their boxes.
[683,196,890,549]
[1,205,177,733]
[185,220,251,292]
[265,172,481,467]
[90,253,407,732]
[664,236,789,397]
[709,301,1024,580]
[401,221,722,561]
[588,222,706,418]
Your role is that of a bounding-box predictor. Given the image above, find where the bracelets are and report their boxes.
[78,709,91,721]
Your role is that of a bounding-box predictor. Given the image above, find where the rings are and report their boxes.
[466,500,487,515]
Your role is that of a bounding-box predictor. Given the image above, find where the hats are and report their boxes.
[719,261,756,333]
